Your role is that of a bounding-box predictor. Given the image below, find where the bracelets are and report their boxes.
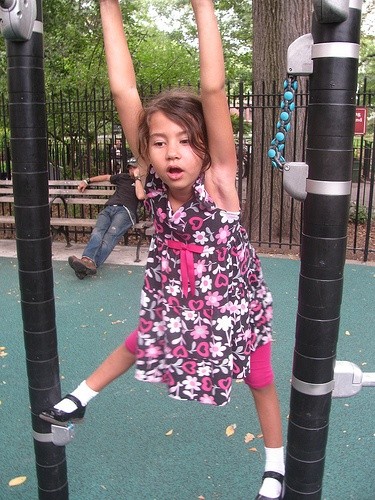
[133,176,142,182]
[86,177,91,184]
[83,179,89,186]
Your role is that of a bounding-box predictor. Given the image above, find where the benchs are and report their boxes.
[0,180,170,262]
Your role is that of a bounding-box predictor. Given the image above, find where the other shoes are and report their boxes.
[68,255,86,279]
[72,256,98,275]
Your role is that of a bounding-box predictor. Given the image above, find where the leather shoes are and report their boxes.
[254,471,286,499]
[40,394,87,425]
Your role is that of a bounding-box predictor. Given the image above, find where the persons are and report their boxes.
[39,0,288,500]
[67,158,148,281]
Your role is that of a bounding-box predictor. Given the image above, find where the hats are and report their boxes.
[126,157,138,168]
